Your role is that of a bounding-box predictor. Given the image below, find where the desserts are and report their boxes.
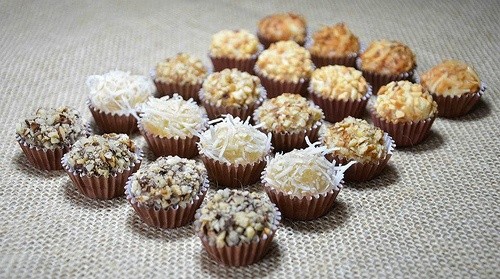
[15,106,281,268]
[89,13,487,221]
